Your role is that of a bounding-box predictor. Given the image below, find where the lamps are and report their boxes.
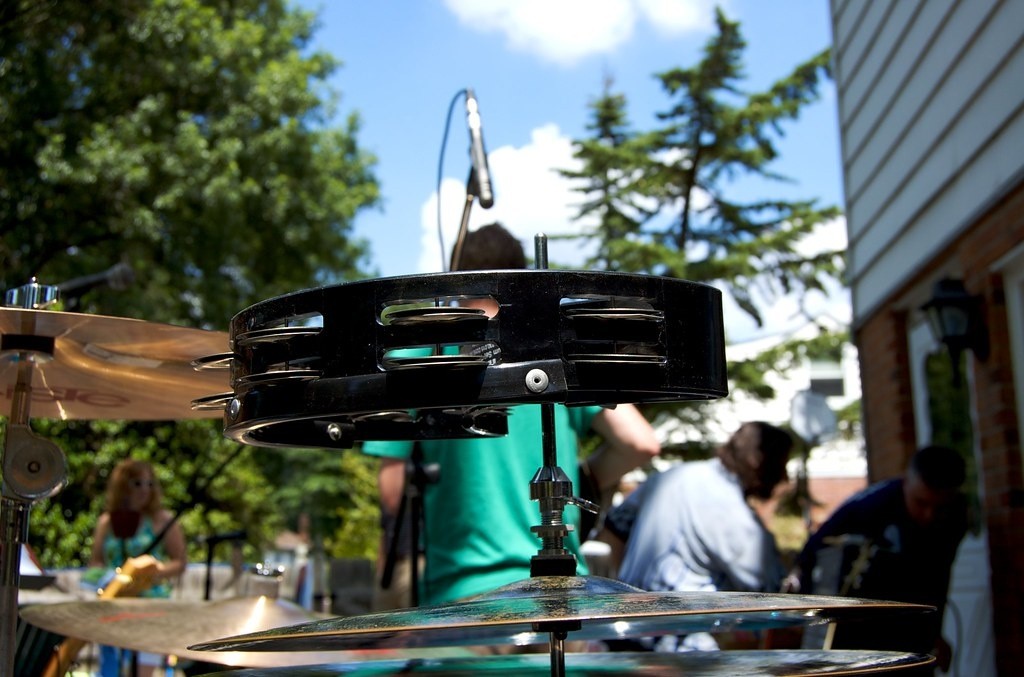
[918,272,992,390]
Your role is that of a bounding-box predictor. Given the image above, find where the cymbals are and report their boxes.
[0,306,314,425]
[19,593,480,668]
[183,573,943,677]
[224,268,731,448]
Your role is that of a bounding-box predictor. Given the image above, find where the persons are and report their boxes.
[88,458,187,677]
[601,422,801,652]
[360,222,661,658]
[798,446,983,677]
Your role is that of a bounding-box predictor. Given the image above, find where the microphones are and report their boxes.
[55,264,135,299]
[205,529,249,547]
[467,90,494,209]
